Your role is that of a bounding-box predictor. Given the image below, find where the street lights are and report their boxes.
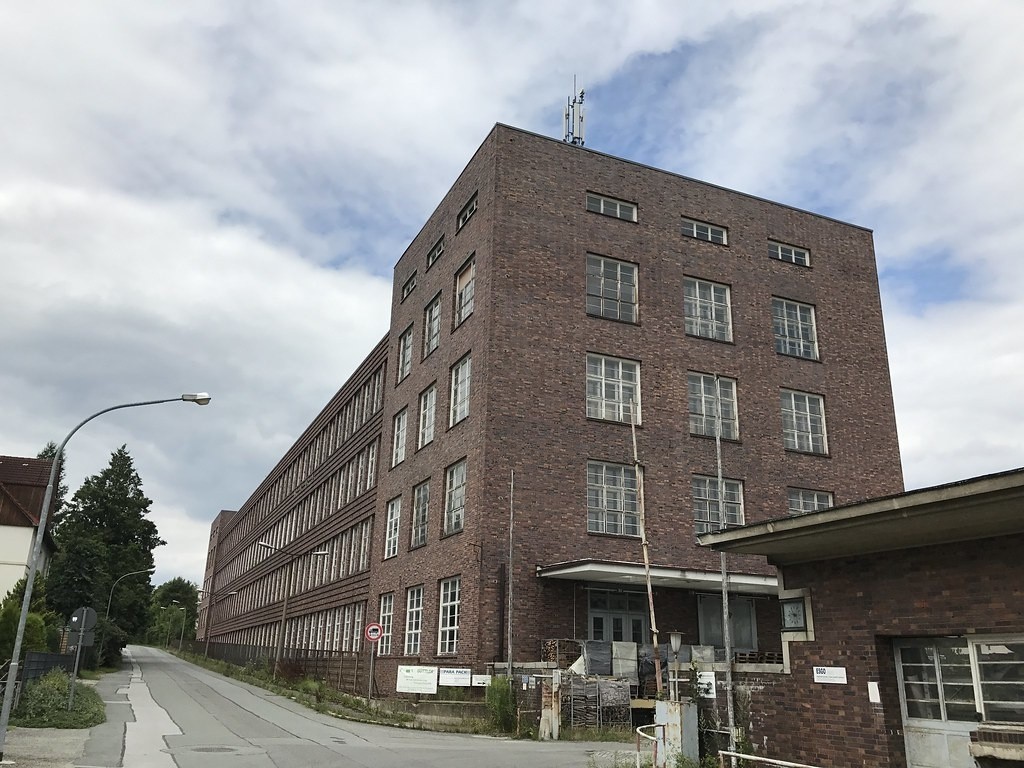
[0,392,211,762]
[97,568,157,671]
[196,589,238,662]
[173,600,203,652]
[161,606,185,648]
[258,541,330,683]
